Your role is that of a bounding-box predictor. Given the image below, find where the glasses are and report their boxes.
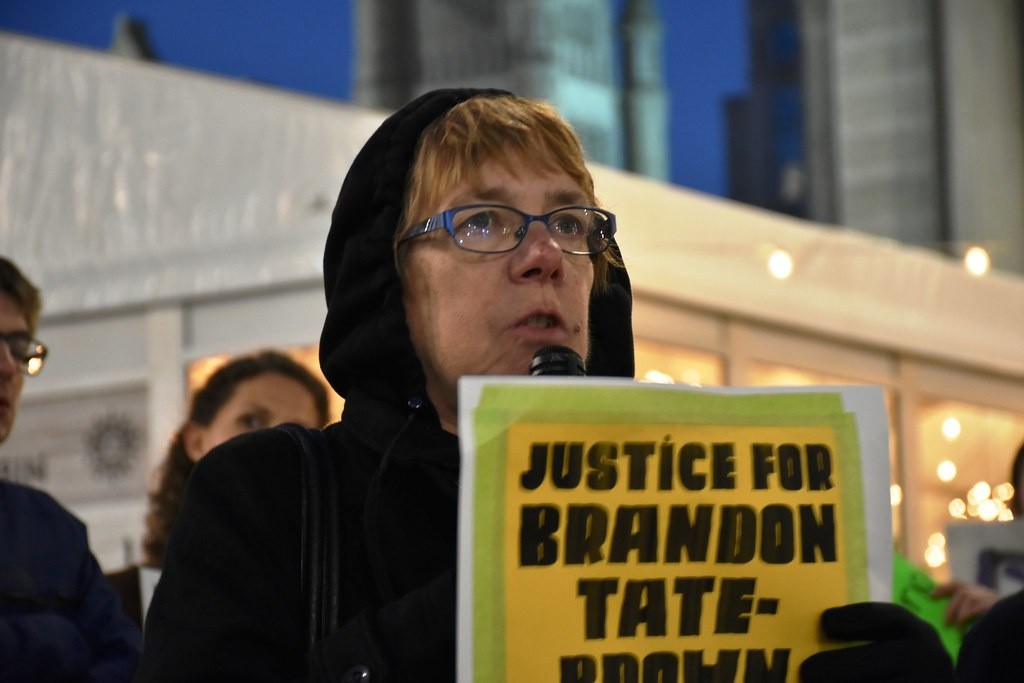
[390,201,621,257]
[1,331,47,376]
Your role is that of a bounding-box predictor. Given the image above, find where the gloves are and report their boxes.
[796,602,962,683]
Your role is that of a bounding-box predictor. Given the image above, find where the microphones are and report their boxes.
[526,344,585,376]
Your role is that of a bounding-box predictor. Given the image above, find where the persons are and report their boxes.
[140,86,955,683]
[888,432,1024,683]
[102,348,330,683]
[0,251,147,683]
